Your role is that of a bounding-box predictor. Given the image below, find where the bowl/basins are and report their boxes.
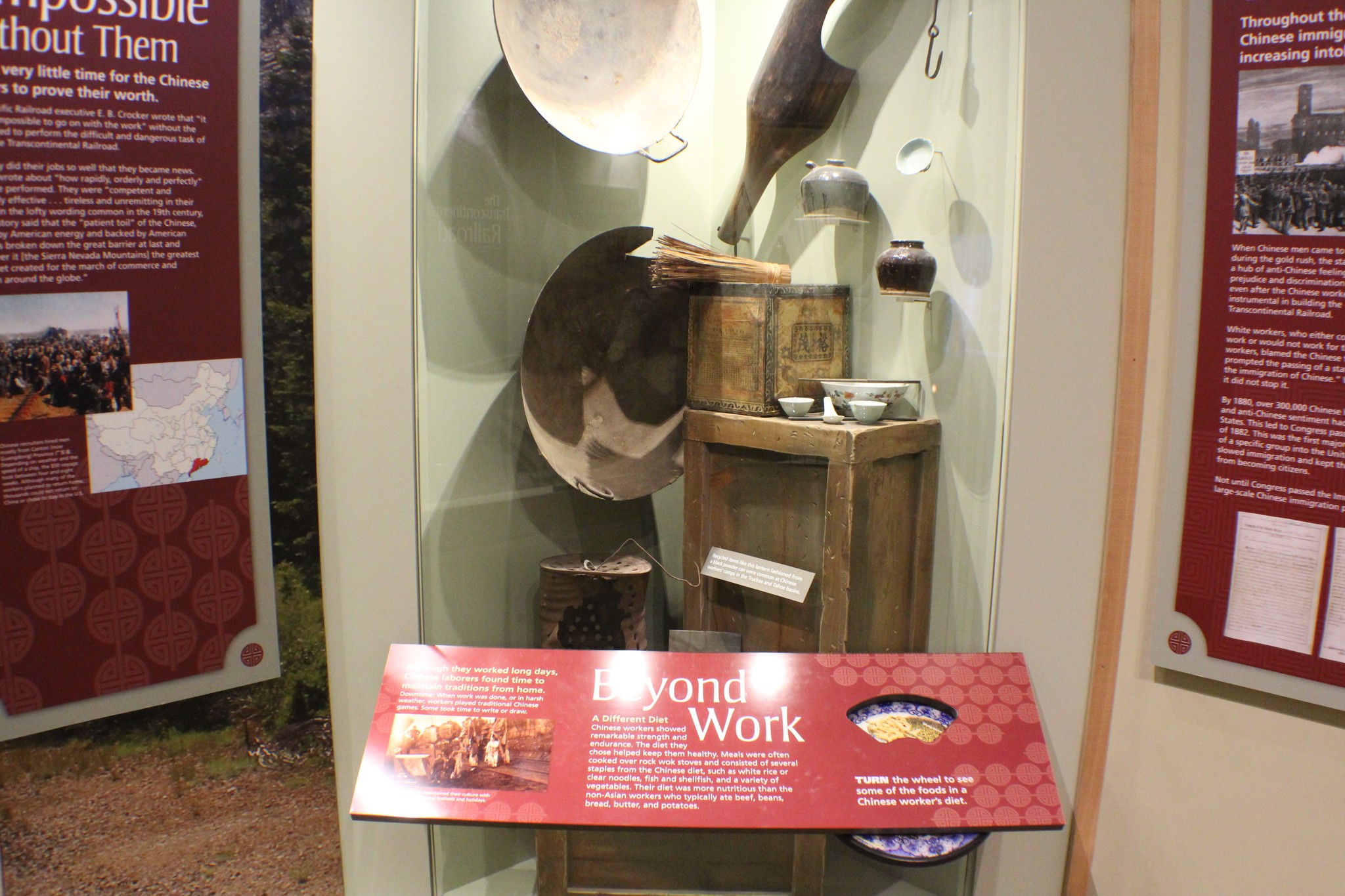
[821,381,910,417]
[848,400,888,425]
[778,398,814,418]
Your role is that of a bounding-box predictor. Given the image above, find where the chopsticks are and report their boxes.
[788,378,921,421]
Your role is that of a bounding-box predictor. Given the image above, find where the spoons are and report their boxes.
[822,396,845,424]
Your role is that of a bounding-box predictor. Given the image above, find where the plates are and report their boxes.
[898,139,935,175]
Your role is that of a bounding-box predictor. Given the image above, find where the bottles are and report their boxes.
[876,239,937,297]
[799,158,870,221]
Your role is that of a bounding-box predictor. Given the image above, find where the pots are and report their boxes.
[490,0,702,163]
[520,224,691,501]
